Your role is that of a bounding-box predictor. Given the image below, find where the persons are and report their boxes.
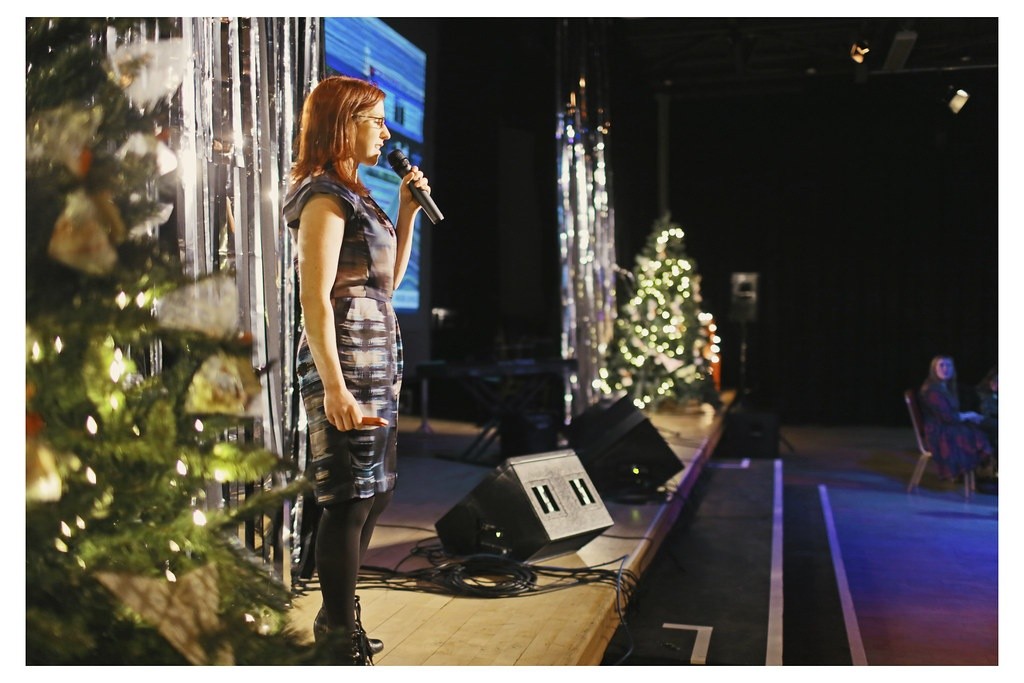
[924,355,992,499]
[277,78,430,666]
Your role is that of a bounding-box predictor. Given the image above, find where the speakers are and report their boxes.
[569,394,685,500]
[433,449,614,567]
[731,271,764,321]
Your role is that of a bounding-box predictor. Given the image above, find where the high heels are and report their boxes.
[313,606,375,666]
[313,593,384,654]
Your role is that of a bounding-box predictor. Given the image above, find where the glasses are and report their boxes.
[352,114,386,128]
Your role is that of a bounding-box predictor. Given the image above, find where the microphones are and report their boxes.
[387,148,445,226]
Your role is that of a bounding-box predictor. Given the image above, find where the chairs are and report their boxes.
[904,390,977,499]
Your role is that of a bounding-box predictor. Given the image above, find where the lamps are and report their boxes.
[850,39,870,64]
